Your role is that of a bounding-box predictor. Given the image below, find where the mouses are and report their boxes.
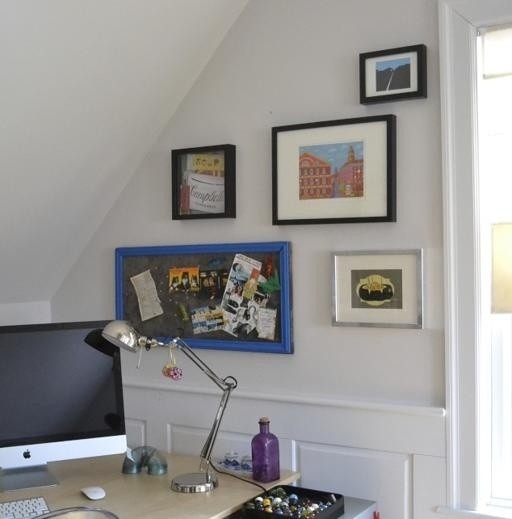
[80,486,106,500]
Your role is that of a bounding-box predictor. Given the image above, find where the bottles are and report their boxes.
[251,417,280,483]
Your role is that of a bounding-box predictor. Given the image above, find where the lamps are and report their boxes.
[98,316,238,493]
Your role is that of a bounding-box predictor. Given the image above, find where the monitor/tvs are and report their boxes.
[0,319,127,491]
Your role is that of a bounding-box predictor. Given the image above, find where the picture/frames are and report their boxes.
[115,241,295,354]
[332,248,423,328]
[272,113,398,225]
[358,44,428,106]
[171,144,237,219]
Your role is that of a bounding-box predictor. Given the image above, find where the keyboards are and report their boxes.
[0,496,51,519]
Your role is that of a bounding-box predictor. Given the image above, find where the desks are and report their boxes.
[4,445,301,519]
